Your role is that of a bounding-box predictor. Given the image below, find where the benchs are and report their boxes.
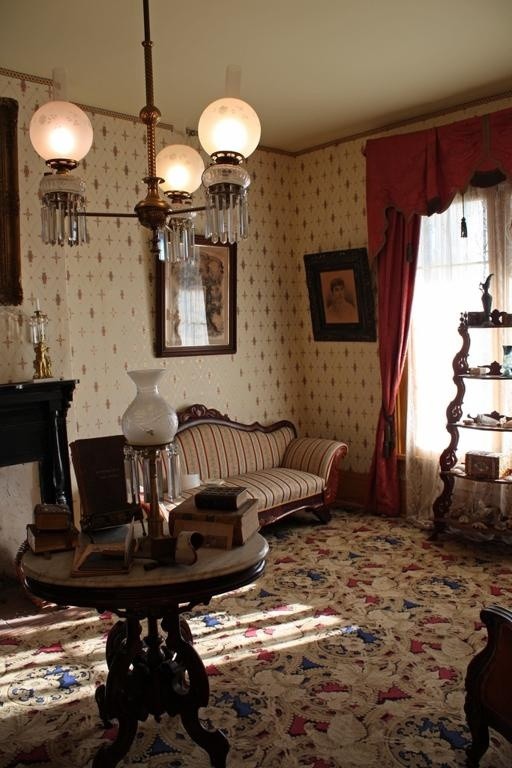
[166,402,351,532]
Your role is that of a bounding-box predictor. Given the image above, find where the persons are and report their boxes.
[326,277,356,325]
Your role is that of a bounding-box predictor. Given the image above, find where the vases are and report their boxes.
[120,368,178,446]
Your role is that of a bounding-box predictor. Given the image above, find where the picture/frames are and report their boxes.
[303,248,377,343]
[154,233,238,357]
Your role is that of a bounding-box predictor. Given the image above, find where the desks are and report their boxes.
[15,532,269,768]
[0,380,74,523]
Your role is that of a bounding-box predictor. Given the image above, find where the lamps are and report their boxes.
[27,2,264,265]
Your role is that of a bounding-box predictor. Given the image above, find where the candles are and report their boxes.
[35,298,40,311]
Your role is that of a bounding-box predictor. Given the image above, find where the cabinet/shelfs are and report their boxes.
[428,310,511,555]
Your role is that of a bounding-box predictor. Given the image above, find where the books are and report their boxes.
[194,486,249,512]
[26,524,79,556]
[70,515,137,577]
[35,504,72,531]
[169,492,261,547]
